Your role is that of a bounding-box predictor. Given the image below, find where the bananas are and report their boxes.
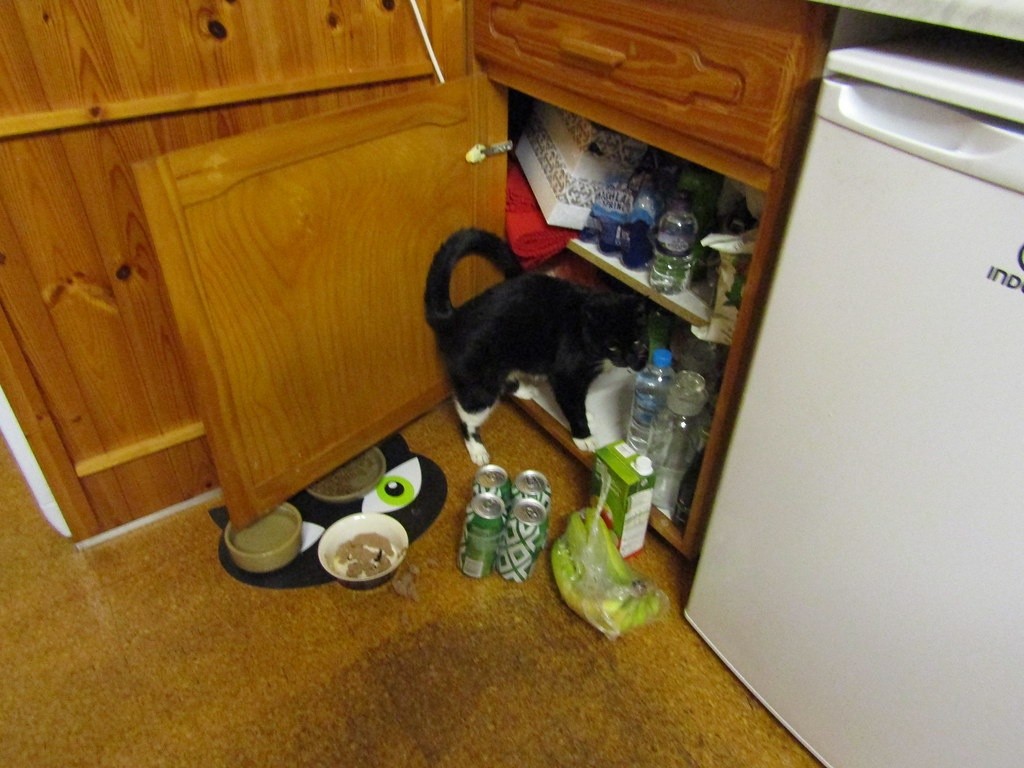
[552,508,663,635]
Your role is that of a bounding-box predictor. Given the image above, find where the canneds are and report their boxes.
[455,464,551,583]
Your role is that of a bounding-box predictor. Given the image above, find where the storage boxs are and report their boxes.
[514,103,649,228]
[537,362,643,450]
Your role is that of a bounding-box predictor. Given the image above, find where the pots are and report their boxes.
[224,501,302,573]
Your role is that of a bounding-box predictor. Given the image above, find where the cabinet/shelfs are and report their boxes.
[1,0,841,557]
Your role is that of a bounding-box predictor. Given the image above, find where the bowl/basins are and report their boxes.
[317,513,409,589]
[305,445,387,503]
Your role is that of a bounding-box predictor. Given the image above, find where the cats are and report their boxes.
[423,227,647,468]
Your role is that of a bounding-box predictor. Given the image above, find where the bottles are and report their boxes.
[675,163,725,283]
[647,305,674,363]
[626,348,676,453]
[646,190,698,294]
[648,370,711,509]
